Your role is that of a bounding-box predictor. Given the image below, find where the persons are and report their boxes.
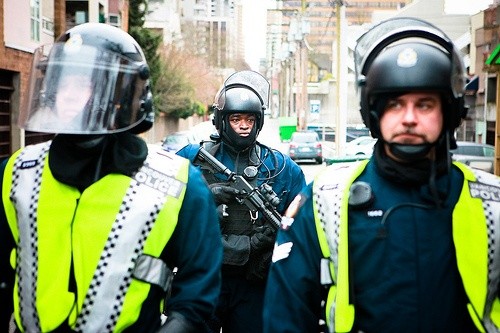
[0,21,224,333]
[263,17,500,333]
[174,70,307,333]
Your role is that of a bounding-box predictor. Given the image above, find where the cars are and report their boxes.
[160,120,217,154]
[289,131,322,164]
[449,141,495,176]
[316,129,379,160]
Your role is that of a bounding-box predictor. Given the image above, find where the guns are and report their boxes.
[190,147,283,231]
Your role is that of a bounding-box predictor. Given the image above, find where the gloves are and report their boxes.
[251,225,276,252]
[208,182,239,205]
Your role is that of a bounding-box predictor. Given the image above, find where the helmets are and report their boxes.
[36,22,155,134]
[360,34,465,160]
[212,83,266,155]
